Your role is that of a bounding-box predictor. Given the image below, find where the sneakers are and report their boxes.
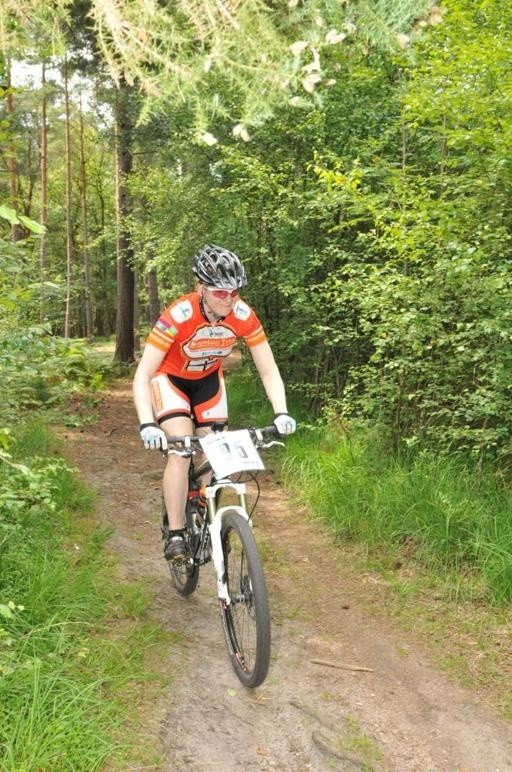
[162,532,189,562]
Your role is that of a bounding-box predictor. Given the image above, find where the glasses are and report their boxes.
[201,284,242,299]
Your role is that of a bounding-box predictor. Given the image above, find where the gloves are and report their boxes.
[271,413,296,441]
[138,422,169,452]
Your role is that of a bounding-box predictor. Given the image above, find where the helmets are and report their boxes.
[191,242,248,292]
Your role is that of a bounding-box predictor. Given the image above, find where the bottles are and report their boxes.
[193,483,210,529]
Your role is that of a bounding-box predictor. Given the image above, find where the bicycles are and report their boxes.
[148,425,288,688]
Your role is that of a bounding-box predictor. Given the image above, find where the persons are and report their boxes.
[132,243,297,562]
